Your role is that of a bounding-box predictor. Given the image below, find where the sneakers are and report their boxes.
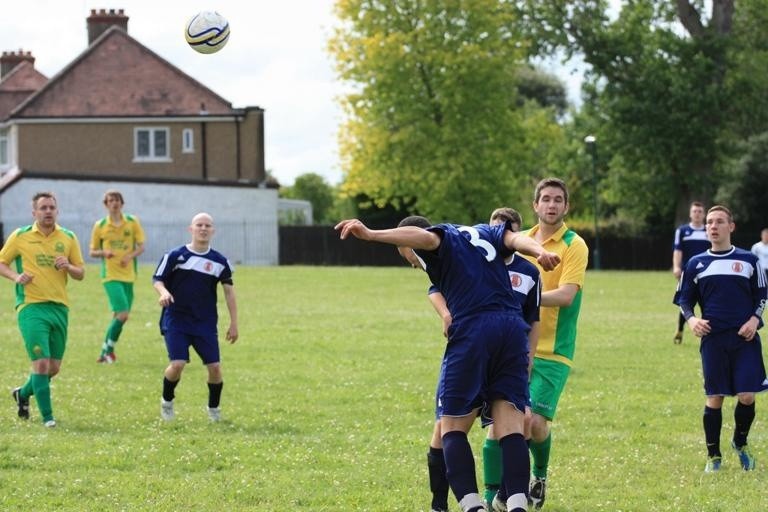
[207,406,222,422]
[674,327,684,344]
[12,386,30,420]
[730,438,757,471]
[96,353,118,364]
[492,491,507,512]
[528,476,545,509]
[704,454,722,472]
[161,395,174,421]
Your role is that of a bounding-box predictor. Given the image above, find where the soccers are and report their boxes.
[184,8,232,55]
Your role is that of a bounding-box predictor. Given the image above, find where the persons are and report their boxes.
[335,215,561,512]
[482,177,589,512]
[89,190,145,363]
[751,228,768,301]
[674,205,768,472]
[426,207,542,512]
[152,213,238,422]
[0,192,86,428]
[671,201,712,344]
